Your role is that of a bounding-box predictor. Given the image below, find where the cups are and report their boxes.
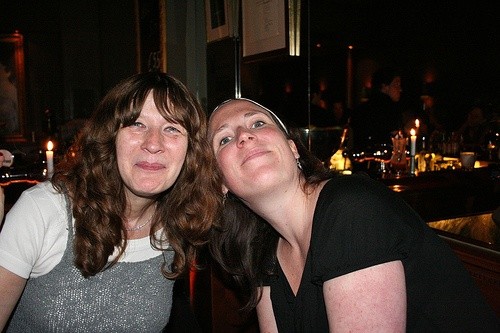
[460,151,476,170]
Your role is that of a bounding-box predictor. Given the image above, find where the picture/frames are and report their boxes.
[135,0,167,75]
[0,31,29,142]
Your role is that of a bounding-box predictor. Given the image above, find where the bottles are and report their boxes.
[29,112,58,171]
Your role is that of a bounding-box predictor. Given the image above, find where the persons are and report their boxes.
[0,68,224,333]
[208,97,500,333]
[456,105,487,145]
[352,67,404,143]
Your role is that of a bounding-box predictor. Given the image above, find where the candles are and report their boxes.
[45,141,54,178]
[410,130,417,156]
